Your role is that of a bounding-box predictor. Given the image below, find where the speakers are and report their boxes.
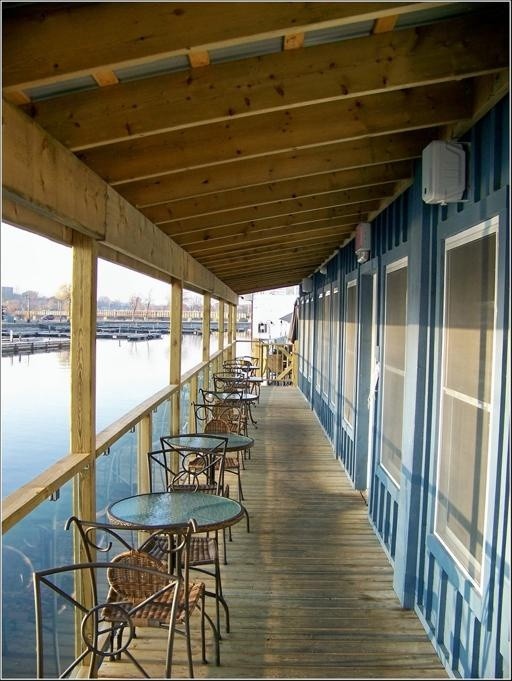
[354,222,371,263]
[302,278,313,292]
[421,139,466,205]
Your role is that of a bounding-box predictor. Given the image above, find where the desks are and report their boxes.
[168,433,254,487]
[105,491,246,668]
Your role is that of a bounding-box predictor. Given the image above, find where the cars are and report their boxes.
[42,315,55,320]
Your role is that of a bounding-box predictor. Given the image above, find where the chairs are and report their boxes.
[32,561,181,679]
[159,431,233,543]
[63,516,206,679]
[138,448,224,638]
[197,357,261,460]
[187,400,244,500]
